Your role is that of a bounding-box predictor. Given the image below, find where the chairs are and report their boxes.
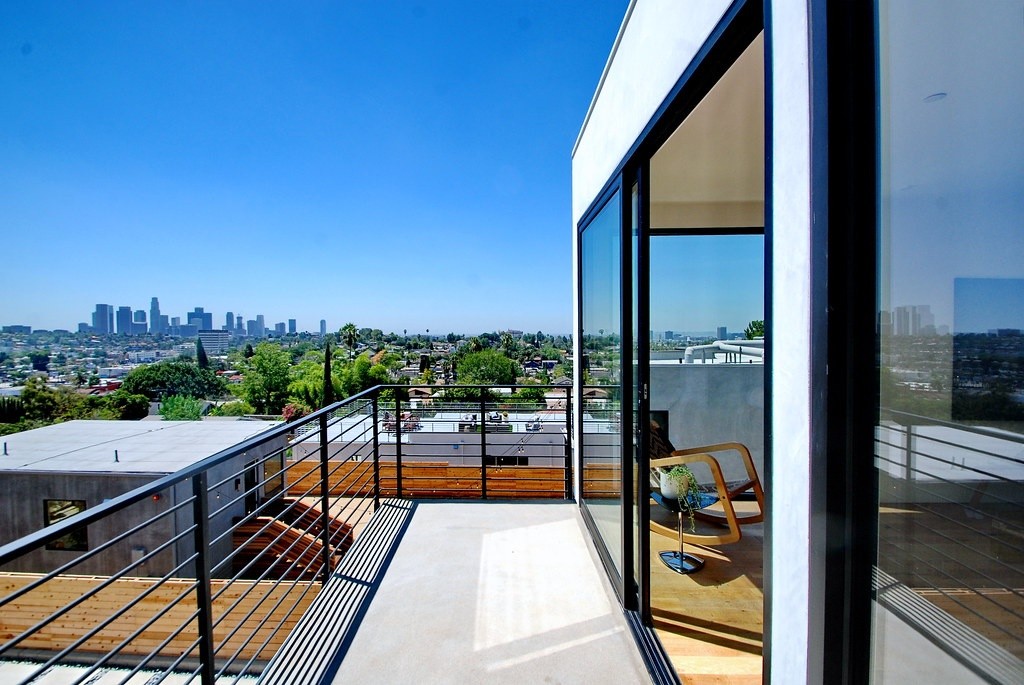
[651,418,764,545]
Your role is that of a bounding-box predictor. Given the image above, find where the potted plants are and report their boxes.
[656,465,701,531]
[503,411,509,421]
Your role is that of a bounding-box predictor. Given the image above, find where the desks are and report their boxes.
[650,493,720,574]
[459,414,473,432]
[474,423,512,433]
[388,413,413,422]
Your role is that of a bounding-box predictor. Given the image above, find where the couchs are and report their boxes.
[525,423,541,432]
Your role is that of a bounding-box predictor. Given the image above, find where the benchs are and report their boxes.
[402,417,421,429]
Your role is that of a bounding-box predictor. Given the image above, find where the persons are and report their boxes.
[528,418,543,428]
[503,413,509,423]
[468,414,475,429]
